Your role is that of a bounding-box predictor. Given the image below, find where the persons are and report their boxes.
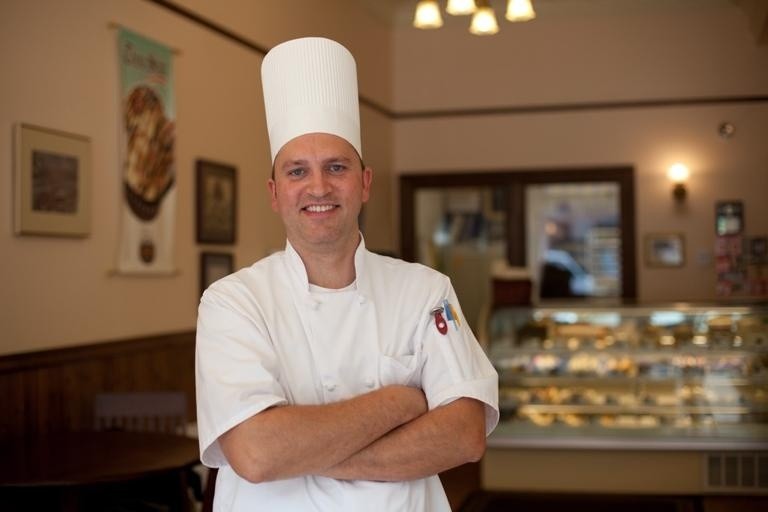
[194,133,500,512]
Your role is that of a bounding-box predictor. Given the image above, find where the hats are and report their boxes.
[260,36,362,165]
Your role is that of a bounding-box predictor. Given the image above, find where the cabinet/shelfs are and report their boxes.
[478,304,766,492]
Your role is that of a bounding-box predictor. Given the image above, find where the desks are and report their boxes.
[1,428,198,512]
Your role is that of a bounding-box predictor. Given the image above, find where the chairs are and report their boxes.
[93,390,189,434]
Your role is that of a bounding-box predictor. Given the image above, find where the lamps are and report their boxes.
[668,163,691,200]
[411,0,536,37]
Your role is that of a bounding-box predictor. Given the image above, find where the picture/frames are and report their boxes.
[713,200,744,238]
[192,159,241,247]
[201,255,234,294]
[641,230,688,268]
[13,122,96,240]
